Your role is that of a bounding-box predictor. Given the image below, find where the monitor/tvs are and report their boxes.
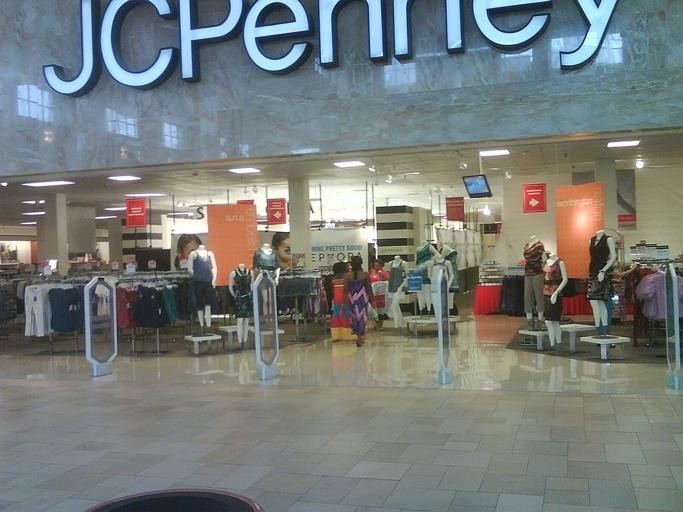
[462,174,492,198]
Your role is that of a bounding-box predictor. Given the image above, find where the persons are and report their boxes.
[585,228,617,327]
[187,245,217,328]
[253,243,281,321]
[522,235,547,321]
[385,242,459,329]
[541,252,569,346]
[173,234,202,272]
[272,232,292,270]
[604,262,637,322]
[325,255,389,348]
[229,263,255,344]
